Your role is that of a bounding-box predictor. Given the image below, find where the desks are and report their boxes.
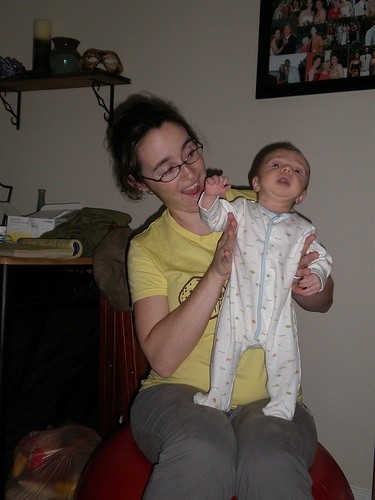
[0,254,94,351]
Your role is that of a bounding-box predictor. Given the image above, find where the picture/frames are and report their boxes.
[254,0,375,99]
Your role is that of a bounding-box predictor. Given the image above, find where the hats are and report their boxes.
[93,227,134,312]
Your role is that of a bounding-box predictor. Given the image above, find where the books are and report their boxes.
[0,236,83,260]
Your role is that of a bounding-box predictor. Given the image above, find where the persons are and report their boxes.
[193,141,334,421]
[267,0,375,84]
[102,90,319,499]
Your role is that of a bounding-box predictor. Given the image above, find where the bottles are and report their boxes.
[37,189,46,212]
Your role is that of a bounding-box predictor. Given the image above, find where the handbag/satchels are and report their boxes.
[5,424,98,499]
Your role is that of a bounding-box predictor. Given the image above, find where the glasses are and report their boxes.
[142,138,203,183]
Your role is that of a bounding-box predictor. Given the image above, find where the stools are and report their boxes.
[72,417,358,499]
